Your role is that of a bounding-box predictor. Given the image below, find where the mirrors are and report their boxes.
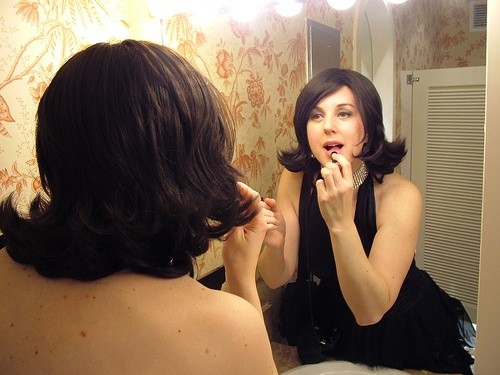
[1,0,487,374]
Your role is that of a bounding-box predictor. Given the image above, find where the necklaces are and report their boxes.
[348,161,367,190]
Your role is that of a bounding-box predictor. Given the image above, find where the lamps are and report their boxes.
[148,0,407,27]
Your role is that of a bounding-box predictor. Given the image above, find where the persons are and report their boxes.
[0,38,279,375]
[254,68,475,375]
